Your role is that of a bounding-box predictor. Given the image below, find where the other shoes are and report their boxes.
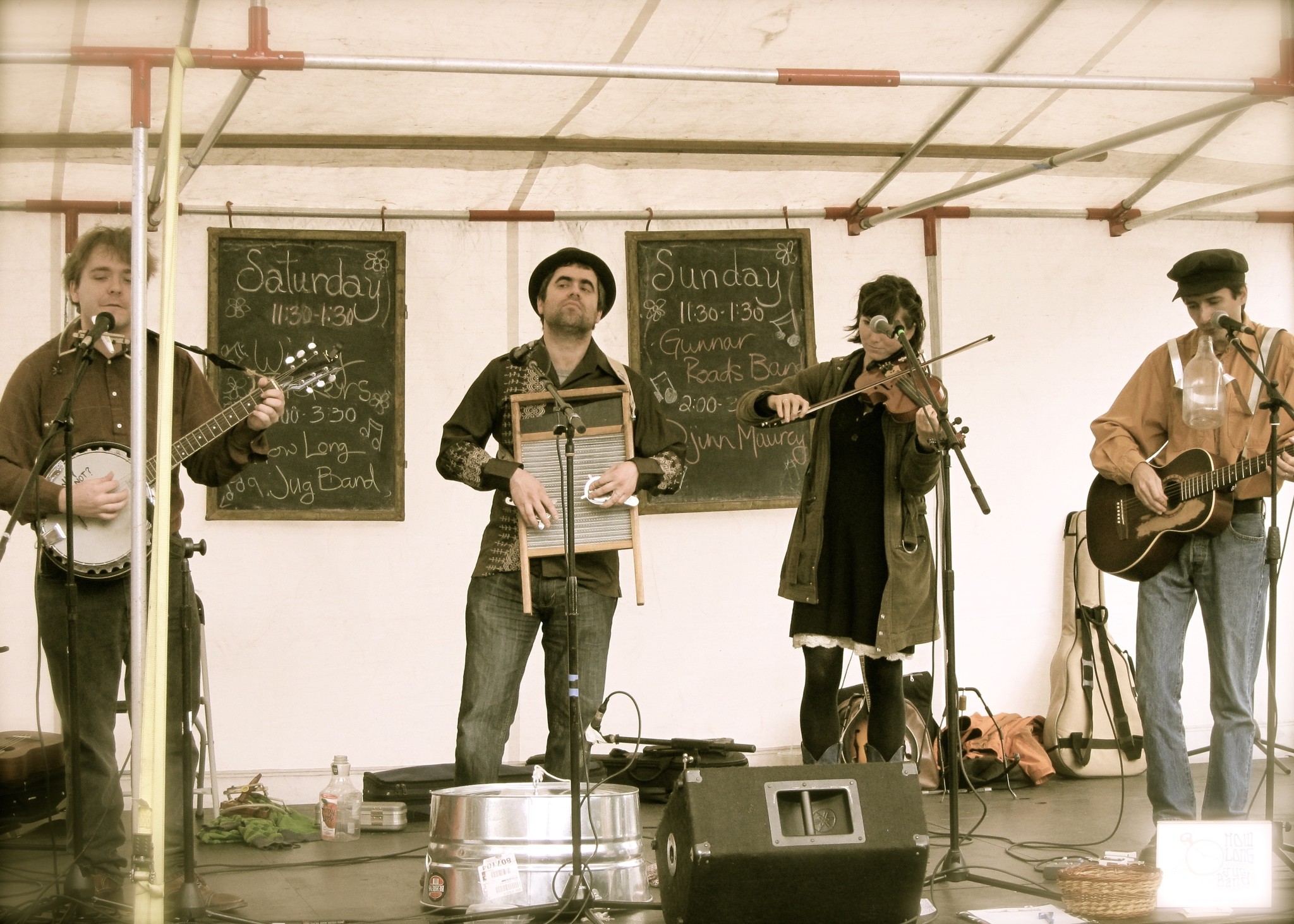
[93,873,125,905]
[164,867,248,919]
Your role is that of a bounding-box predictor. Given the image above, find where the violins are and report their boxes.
[854,359,967,449]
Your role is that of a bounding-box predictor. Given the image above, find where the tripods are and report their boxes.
[1188,719,1294,775]
[899,331,1060,904]
[1,317,250,924]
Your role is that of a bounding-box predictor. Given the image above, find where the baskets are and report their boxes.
[1056,864,1163,919]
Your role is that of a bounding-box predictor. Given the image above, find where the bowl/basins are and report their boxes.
[464,904,535,924]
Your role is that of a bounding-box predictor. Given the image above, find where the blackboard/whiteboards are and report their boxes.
[205,227,406,523]
[624,228,819,516]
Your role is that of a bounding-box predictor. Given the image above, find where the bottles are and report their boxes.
[1182,335,1225,431]
[319,755,362,842]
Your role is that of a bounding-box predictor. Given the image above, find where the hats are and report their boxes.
[1168,248,1248,303]
[529,248,616,320]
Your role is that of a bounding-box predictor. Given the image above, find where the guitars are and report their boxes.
[30,342,347,580]
[1084,442,1294,582]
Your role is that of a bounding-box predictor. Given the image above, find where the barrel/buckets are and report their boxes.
[420,782,654,909]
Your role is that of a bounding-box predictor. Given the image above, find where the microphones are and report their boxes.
[1212,310,1254,336]
[509,339,538,367]
[869,315,901,342]
[580,698,608,754]
[80,311,116,350]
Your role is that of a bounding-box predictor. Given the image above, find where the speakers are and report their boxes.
[650,759,924,924]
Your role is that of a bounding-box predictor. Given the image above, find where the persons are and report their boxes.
[1090,248,1294,864]
[436,247,686,788]
[0,225,285,911]
[738,279,949,766]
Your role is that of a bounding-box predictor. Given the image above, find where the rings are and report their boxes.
[1283,472,1288,478]
[782,399,788,404]
[924,421,929,424]
[614,492,621,498]
